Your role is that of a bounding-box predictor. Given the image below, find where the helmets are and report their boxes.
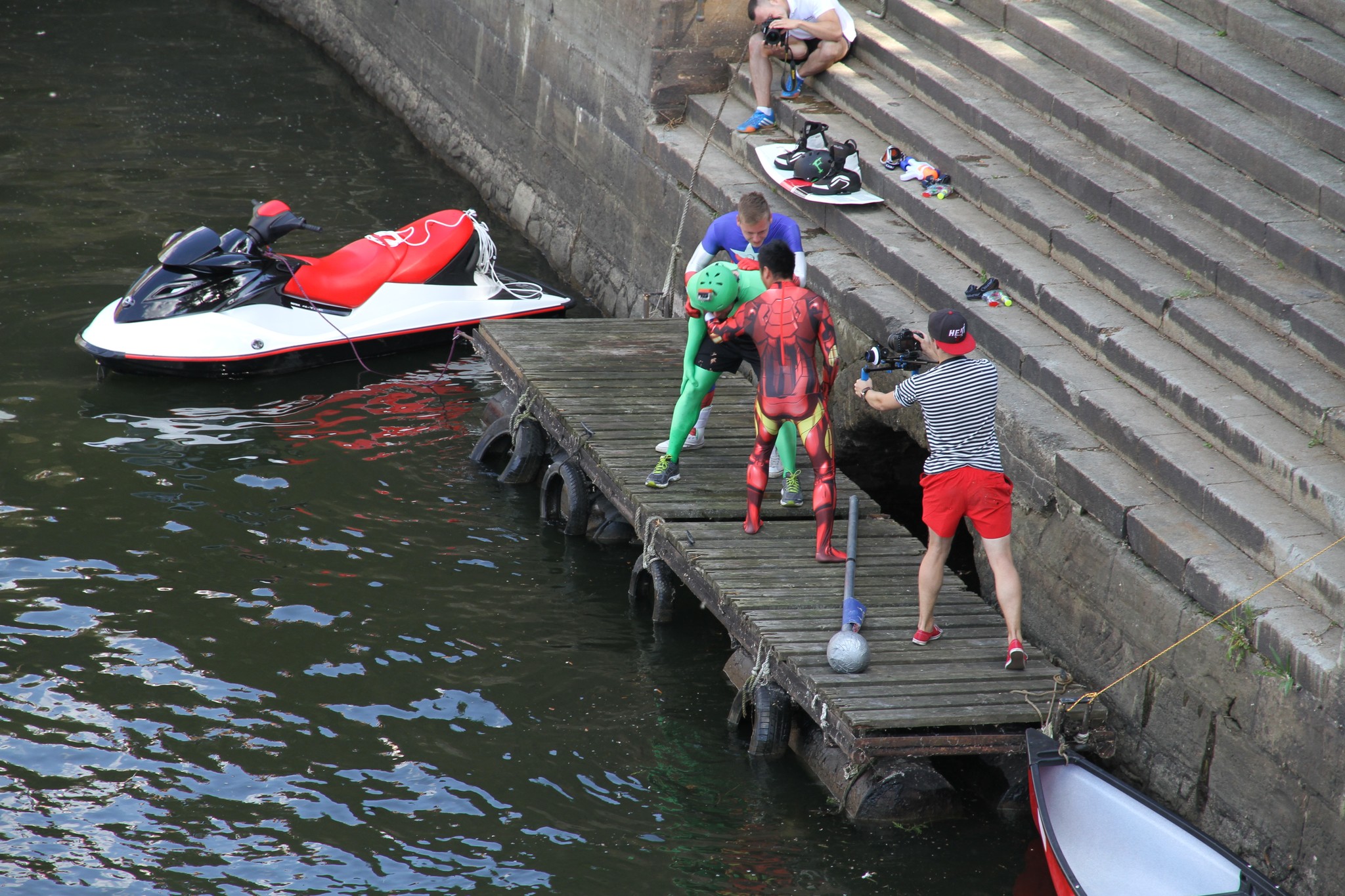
[793,149,833,182]
[696,265,738,312]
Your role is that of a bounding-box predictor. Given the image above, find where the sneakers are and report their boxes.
[736,109,776,133]
[768,445,784,478]
[811,142,862,195]
[912,624,943,646]
[1005,639,1028,670]
[774,120,829,171]
[780,69,805,99]
[645,454,681,488]
[780,466,803,507]
[655,427,704,453]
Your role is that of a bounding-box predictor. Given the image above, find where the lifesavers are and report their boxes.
[469,409,552,490]
[725,673,791,758]
[537,458,590,538]
[627,549,679,626]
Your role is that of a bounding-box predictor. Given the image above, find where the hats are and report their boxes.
[928,308,976,355]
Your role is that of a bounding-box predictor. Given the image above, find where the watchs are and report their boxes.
[860,387,873,403]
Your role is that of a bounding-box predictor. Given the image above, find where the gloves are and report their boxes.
[684,271,701,318]
[735,254,760,271]
[680,361,699,394]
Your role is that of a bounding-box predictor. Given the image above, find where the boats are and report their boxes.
[1026,727,1293,896]
[74,199,577,380]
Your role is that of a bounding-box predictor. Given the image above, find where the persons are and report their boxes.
[854,308,1028,670]
[644,261,805,509]
[736,0,857,134]
[702,239,848,563]
[655,192,807,478]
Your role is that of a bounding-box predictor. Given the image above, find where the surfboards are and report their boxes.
[754,144,886,204]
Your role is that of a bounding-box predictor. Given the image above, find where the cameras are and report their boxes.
[760,17,785,45]
[886,329,924,356]
